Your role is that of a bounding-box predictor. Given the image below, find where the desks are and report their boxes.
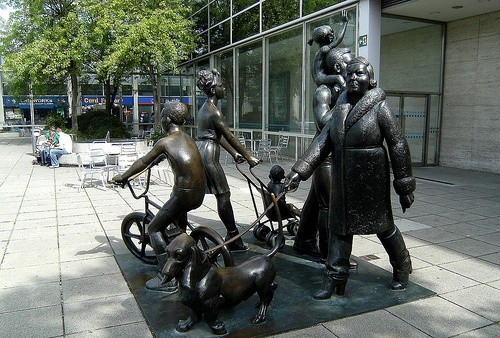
[246,139,261,159]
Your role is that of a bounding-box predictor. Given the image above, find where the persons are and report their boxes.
[41,125,60,167]
[112,101,206,292]
[268,165,302,217]
[308,10,347,91]
[291,47,359,268]
[176,67,260,251]
[49,128,72,167]
[285,57,417,299]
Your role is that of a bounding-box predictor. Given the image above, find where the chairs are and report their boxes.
[76,129,173,193]
[226,135,289,166]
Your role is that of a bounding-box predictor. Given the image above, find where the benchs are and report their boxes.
[57,143,90,167]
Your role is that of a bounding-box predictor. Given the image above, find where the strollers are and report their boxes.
[236,159,300,246]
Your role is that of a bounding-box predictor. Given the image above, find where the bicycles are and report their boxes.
[108,162,232,269]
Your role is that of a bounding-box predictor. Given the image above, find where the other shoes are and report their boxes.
[48,166,59,169]
